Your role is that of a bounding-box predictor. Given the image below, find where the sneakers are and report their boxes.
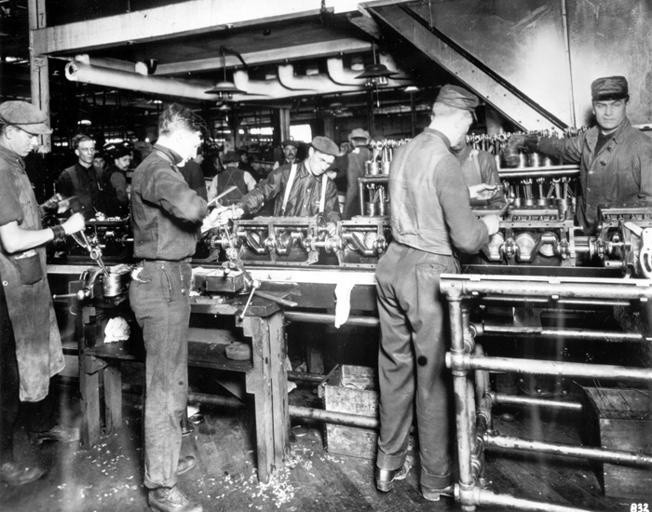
[147,485,205,512]
[420,483,455,503]
[375,458,414,492]
[0,460,48,487]
[176,456,195,477]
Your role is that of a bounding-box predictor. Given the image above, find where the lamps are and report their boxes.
[205,45,247,122]
[354,40,399,108]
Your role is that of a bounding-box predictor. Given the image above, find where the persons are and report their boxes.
[0,75,652,511]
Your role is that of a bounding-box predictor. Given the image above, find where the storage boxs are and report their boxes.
[571,377,652,503]
[317,363,378,461]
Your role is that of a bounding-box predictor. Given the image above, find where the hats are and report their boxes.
[346,127,369,140]
[0,100,52,135]
[223,140,299,164]
[435,83,480,122]
[311,135,339,158]
[590,75,629,102]
